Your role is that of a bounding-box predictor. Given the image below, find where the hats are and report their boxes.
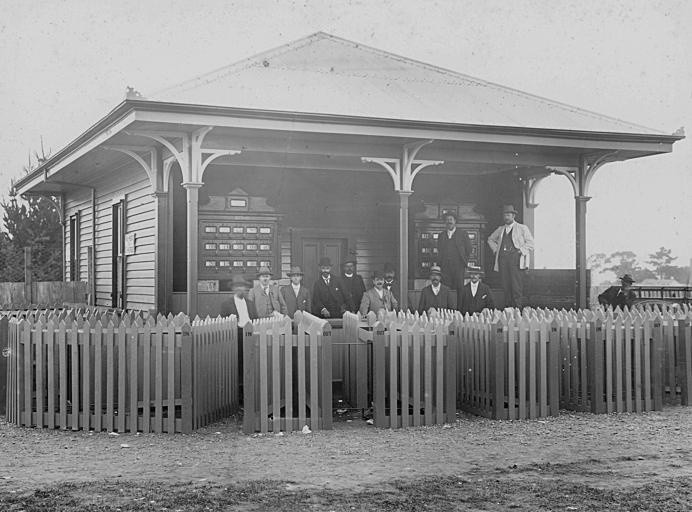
[341,256,357,266]
[229,274,252,286]
[467,267,484,274]
[620,274,635,282]
[287,266,304,275]
[318,258,334,267]
[371,262,395,278]
[256,266,274,277]
[442,211,458,219]
[429,266,442,275]
[501,205,518,214]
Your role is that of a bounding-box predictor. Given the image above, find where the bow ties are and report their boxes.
[385,283,393,286]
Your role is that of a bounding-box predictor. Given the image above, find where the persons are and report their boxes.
[247,266,289,319]
[309,257,347,318]
[357,271,400,315]
[337,258,366,316]
[419,263,453,313]
[437,211,473,290]
[458,265,495,316]
[381,261,401,303]
[279,265,312,318]
[595,273,636,311]
[220,275,258,407]
[486,203,536,309]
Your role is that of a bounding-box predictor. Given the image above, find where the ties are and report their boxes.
[264,287,268,295]
[326,279,328,284]
